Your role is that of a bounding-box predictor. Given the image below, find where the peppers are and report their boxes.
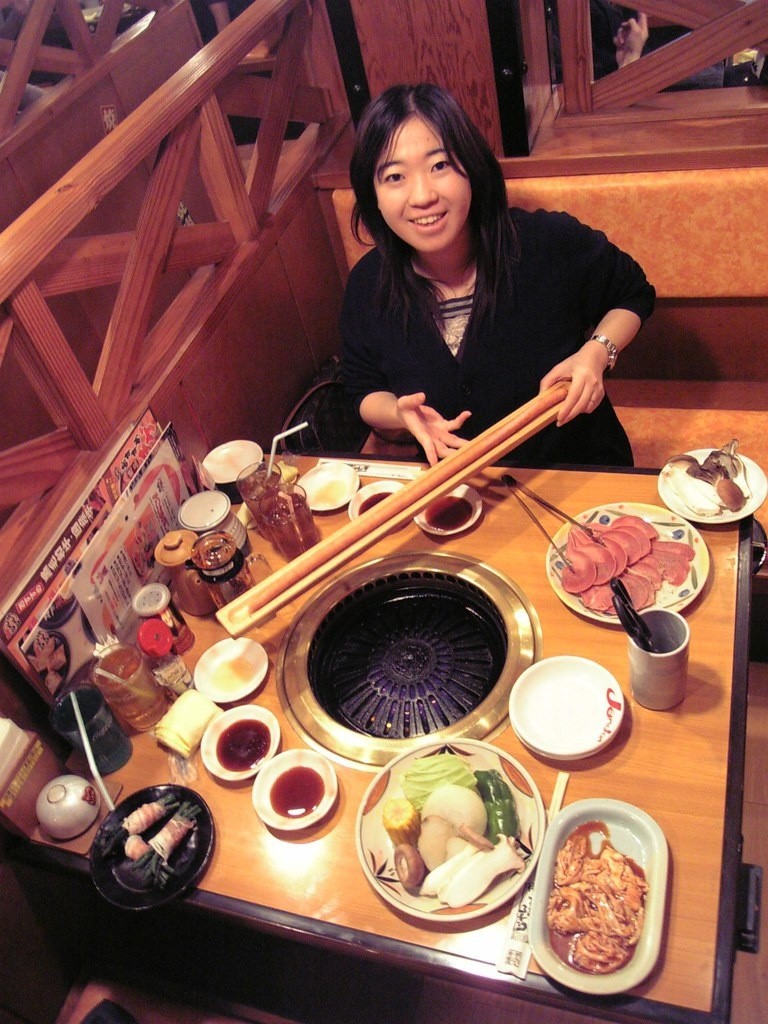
[473,770,519,844]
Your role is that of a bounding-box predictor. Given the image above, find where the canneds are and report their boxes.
[131,582,196,657]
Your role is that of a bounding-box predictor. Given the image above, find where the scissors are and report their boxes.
[608,574,661,653]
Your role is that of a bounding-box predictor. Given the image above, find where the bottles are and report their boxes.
[136,621,196,702]
[132,584,196,653]
[154,490,267,616]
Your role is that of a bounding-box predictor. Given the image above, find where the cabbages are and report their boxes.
[404,753,479,816]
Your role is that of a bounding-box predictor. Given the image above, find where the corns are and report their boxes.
[382,799,421,848]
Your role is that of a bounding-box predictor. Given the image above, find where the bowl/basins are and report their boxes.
[201,439,263,495]
[36,775,101,840]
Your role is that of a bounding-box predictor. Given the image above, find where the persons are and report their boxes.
[614,12,649,70]
[339,82,656,471]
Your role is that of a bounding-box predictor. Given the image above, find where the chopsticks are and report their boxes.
[549,772,570,822]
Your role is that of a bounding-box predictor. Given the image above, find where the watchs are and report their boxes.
[591,334,618,372]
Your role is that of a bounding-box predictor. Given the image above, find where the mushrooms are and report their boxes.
[664,438,753,517]
[394,785,527,909]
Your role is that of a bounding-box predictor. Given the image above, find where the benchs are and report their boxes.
[330,167,768,595]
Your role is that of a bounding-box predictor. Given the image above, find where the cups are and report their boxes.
[629,608,690,711]
[259,482,323,564]
[50,683,132,778]
[89,642,167,733]
[236,461,286,542]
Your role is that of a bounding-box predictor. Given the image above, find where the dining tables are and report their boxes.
[28,453,768,1024]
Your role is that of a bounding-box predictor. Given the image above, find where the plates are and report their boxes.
[545,502,711,624]
[657,449,767,524]
[293,462,360,512]
[508,655,625,762]
[413,483,483,535]
[90,783,215,911]
[528,797,670,996]
[201,705,281,781]
[193,637,268,703]
[355,739,546,921]
[252,750,338,830]
[347,480,405,524]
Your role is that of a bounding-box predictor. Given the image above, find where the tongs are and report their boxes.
[502,473,605,572]
